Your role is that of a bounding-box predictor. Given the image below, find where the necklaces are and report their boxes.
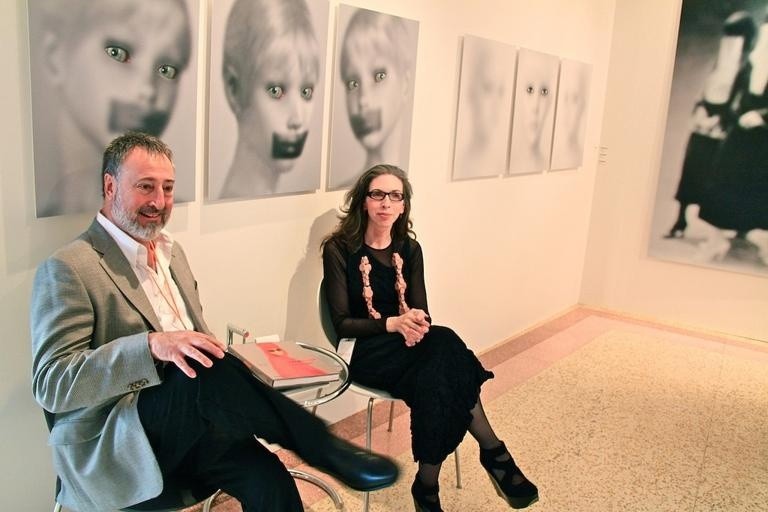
[137,240,187,330]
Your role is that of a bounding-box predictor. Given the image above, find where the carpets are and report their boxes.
[306,325,768,512]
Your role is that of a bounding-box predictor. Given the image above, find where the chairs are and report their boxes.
[43,405,217,512]
[311,277,464,512]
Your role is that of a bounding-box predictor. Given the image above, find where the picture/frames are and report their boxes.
[636,0,768,279]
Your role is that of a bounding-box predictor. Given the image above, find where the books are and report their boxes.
[228,339,341,389]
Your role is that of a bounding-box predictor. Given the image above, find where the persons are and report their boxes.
[698,15,768,238]
[337,8,411,187]
[513,53,555,174]
[36,0,195,218]
[663,11,757,238]
[319,164,540,511]
[217,0,320,198]
[29,131,399,511]
[256,343,326,379]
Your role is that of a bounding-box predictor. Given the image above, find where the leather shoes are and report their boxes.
[298,433,403,493]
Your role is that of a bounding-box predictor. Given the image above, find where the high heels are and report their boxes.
[662,217,689,241]
[478,440,541,511]
[409,471,445,512]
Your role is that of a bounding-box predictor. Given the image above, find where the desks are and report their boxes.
[202,323,352,512]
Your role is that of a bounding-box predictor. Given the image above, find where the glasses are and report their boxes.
[365,188,407,203]
